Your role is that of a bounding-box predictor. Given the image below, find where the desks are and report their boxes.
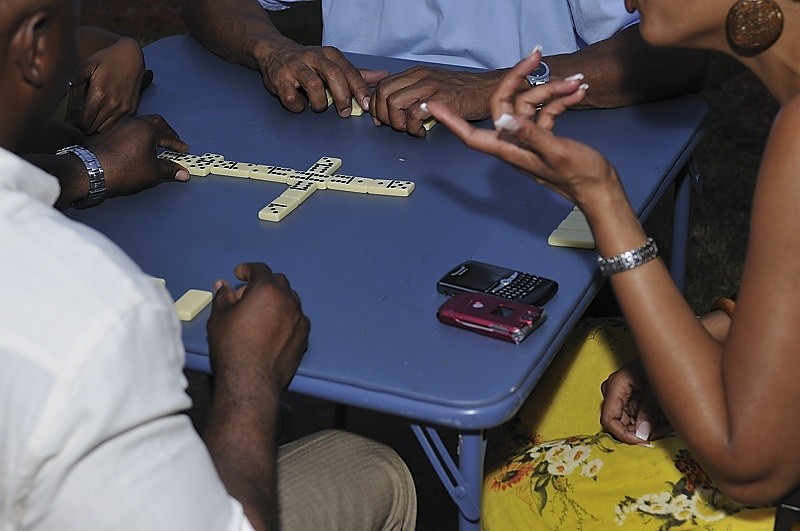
[6,34,713,531]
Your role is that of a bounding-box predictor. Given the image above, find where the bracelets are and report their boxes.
[598,239,660,279]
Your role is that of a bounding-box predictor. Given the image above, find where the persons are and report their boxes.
[177,0,712,140]
[0,1,417,531]
[427,0,800,530]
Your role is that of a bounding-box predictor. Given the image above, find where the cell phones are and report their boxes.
[437,260,558,306]
[437,292,547,344]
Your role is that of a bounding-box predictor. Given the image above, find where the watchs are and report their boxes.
[525,60,551,113]
[55,145,107,209]
